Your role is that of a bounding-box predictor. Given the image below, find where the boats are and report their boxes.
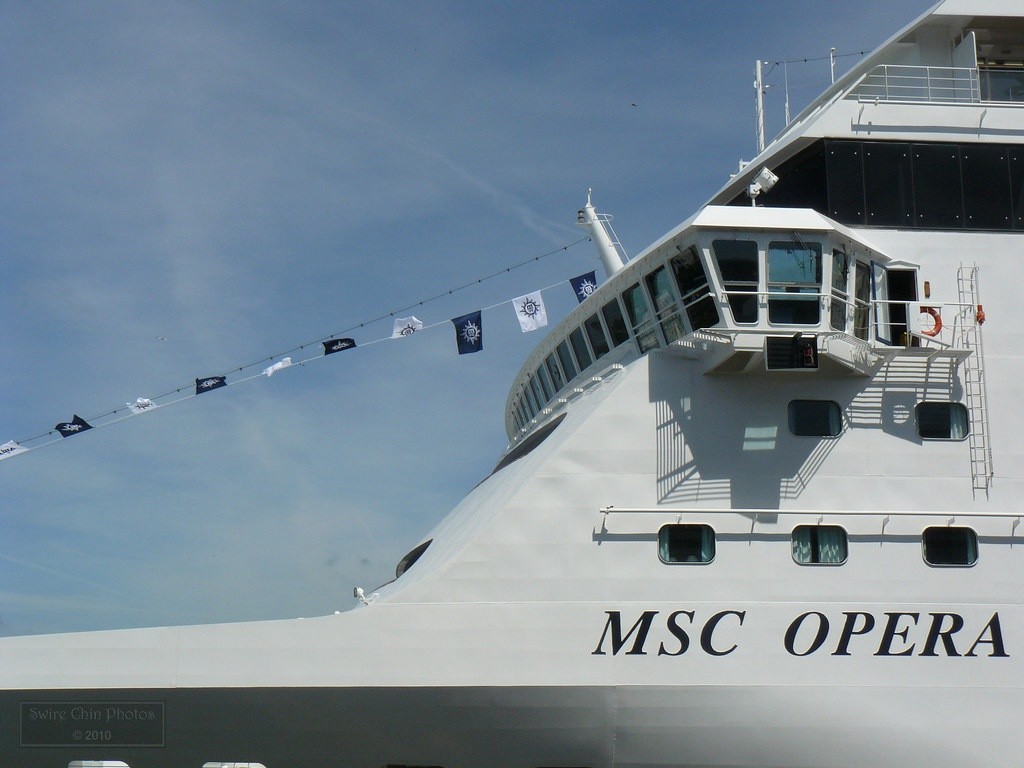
[0,0,1024,768]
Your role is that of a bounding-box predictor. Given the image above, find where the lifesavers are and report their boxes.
[919,306,942,337]
